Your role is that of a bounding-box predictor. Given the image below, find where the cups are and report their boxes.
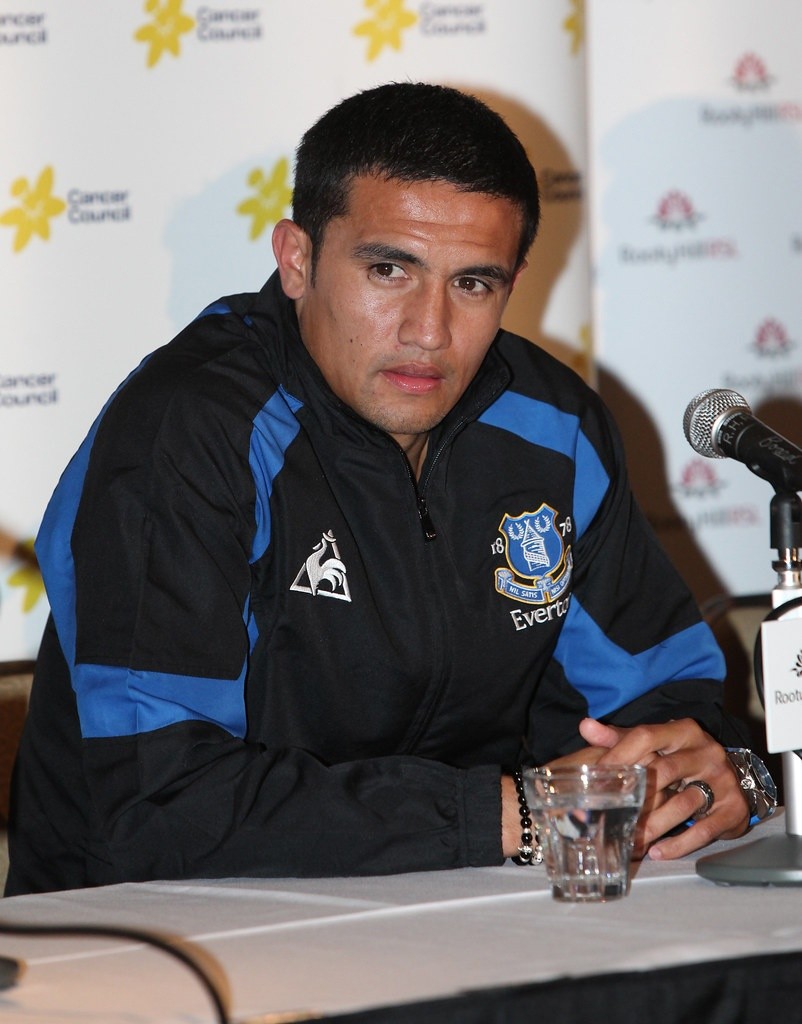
[522,763,646,903]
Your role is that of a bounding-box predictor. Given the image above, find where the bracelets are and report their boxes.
[511,766,555,867]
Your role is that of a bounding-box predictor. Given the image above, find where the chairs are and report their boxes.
[699,594,785,806]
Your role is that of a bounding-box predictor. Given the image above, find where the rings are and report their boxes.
[685,780,713,814]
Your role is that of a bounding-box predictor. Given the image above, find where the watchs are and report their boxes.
[726,745,777,820]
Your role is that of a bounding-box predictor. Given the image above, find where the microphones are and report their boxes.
[683,388,802,492]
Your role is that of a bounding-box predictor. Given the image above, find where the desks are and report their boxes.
[0,808,802,1024]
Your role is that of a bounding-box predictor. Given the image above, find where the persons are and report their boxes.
[7,85,777,895]
